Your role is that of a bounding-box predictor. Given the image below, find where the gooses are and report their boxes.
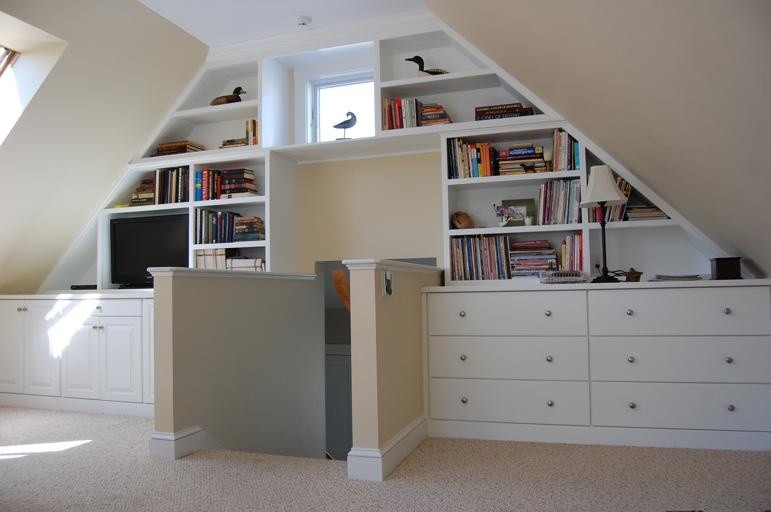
[404,55,449,78]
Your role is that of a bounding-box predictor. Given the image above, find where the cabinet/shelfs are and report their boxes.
[94,61,267,289]
[378,28,748,279]
[0,300,155,404]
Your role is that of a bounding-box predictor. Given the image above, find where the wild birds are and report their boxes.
[334,112,356,138]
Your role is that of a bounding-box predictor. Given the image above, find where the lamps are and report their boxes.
[578,162,630,283]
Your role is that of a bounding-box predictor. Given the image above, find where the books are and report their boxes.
[192,207,266,271]
[651,269,704,282]
[110,117,265,210]
[70,283,96,292]
[446,127,671,281]
[380,89,537,134]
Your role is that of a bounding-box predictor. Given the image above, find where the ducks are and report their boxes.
[210,86,248,105]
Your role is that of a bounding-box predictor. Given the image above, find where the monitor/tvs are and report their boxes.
[110,214,189,289]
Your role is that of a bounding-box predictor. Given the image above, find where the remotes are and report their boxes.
[71,285,97,290]
